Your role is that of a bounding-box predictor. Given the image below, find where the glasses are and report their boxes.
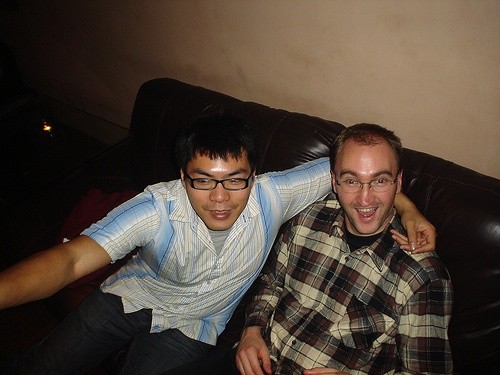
[183,169,254,190]
[335,173,398,193]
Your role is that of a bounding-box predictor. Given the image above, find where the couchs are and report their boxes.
[128,77,499,375]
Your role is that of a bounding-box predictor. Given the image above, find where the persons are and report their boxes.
[191,123,453,375]
[0,114,437,375]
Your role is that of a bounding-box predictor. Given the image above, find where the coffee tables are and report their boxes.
[1,94,129,200]
